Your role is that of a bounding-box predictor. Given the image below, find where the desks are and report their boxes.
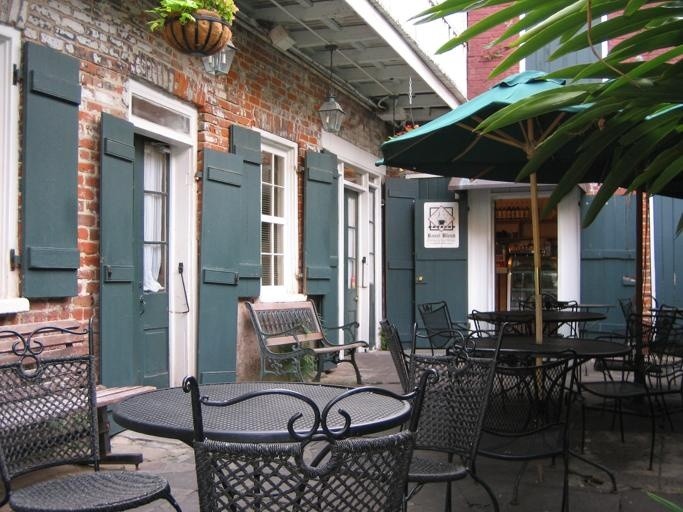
[450,333,638,503]
[110,381,412,512]
[466,308,605,337]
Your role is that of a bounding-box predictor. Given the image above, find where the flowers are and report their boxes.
[387,123,420,139]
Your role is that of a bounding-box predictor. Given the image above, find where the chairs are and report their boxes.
[445,345,580,511]
[182,368,439,512]
[0,315,183,512]
[379,320,424,392]
[580,295,682,472]
[336,320,510,511]
[514,290,580,308]
[417,300,473,358]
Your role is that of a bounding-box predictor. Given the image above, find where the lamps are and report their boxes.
[200,38,239,77]
[317,43,346,133]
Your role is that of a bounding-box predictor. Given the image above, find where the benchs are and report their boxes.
[245,299,368,385]
[0,319,158,474]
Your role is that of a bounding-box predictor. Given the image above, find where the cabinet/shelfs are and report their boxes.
[505,255,557,308]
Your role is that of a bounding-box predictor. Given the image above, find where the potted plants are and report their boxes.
[142,0,239,57]
